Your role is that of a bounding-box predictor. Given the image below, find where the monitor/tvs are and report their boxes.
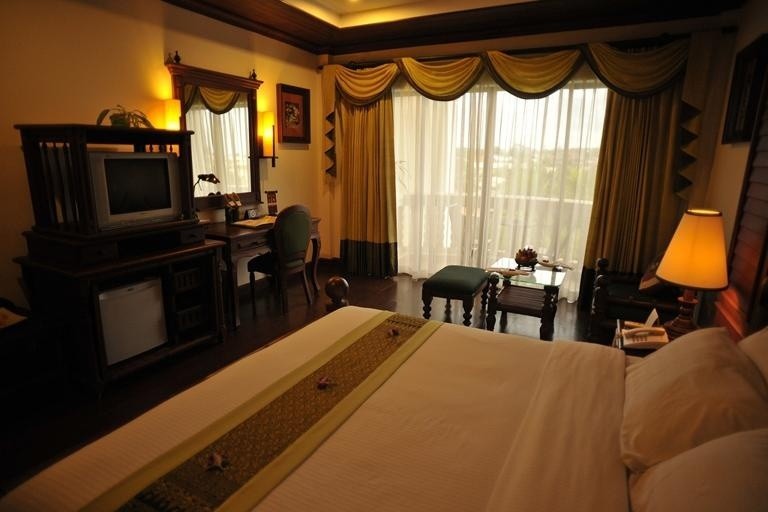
[87,152,182,232]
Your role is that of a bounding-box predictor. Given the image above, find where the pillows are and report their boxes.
[637,248,670,292]
[736,325,767,387]
[628,427,766,511]
[619,325,767,474]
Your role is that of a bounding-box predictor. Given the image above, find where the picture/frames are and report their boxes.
[720,32,767,145]
[276,83,310,144]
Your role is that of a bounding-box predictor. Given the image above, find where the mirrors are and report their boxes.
[164,62,265,213]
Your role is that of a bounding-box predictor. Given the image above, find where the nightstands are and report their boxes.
[611,318,697,359]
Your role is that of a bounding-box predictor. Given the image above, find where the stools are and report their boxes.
[421,262,487,326]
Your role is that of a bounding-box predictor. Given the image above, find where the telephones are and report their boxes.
[619,326,670,351]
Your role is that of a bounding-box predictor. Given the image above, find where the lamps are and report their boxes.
[163,98,181,129]
[256,110,278,166]
[193,173,220,191]
[655,208,729,340]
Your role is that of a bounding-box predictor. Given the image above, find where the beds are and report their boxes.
[2,275,766,511]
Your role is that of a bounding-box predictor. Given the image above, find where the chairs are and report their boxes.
[247,203,312,319]
[581,256,705,344]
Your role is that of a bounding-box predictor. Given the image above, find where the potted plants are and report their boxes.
[95,104,154,128]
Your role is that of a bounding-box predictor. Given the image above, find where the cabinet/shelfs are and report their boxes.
[9,125,226,391]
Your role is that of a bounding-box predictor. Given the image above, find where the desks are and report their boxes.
[205,214,321,331]
[486,256,568,341]
